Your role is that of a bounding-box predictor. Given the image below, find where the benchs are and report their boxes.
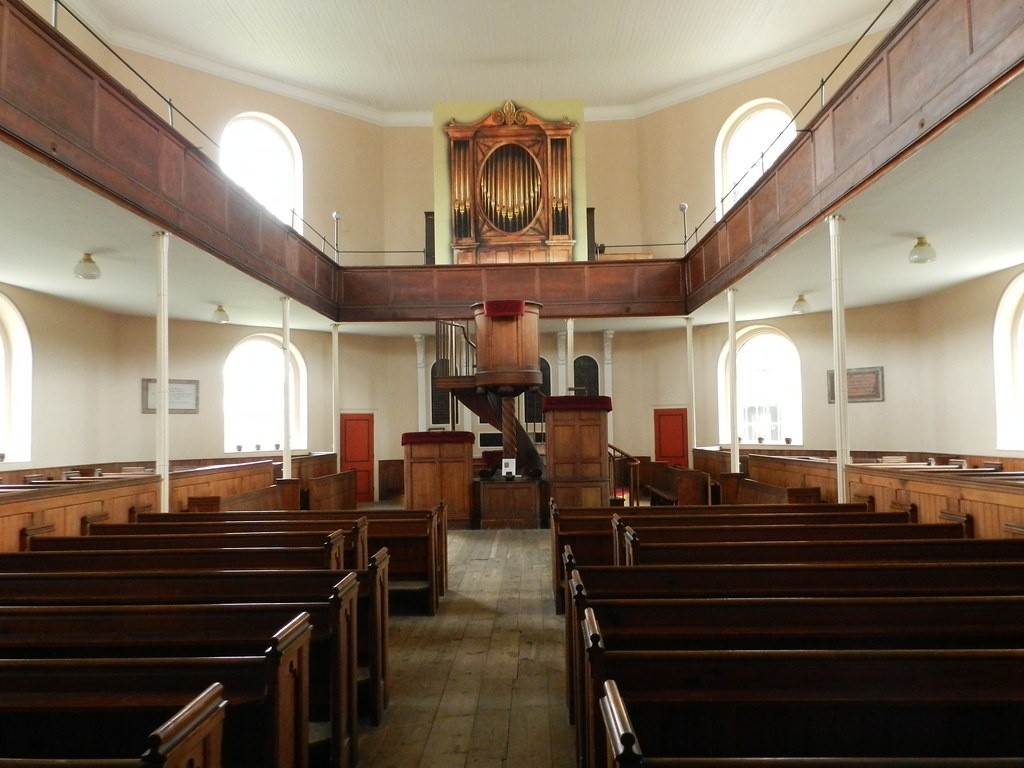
[647,483,677,501]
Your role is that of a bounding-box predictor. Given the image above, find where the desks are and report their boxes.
[599,251,653,260]
[547,495,1024,767]
[0,500,449,768]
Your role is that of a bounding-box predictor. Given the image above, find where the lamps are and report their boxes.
[790,294,812,315]
[72,252,102,280]
[906,238,938,264]
[210,304,231,324]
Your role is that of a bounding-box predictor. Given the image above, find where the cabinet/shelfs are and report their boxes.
[479,478,540,531]
